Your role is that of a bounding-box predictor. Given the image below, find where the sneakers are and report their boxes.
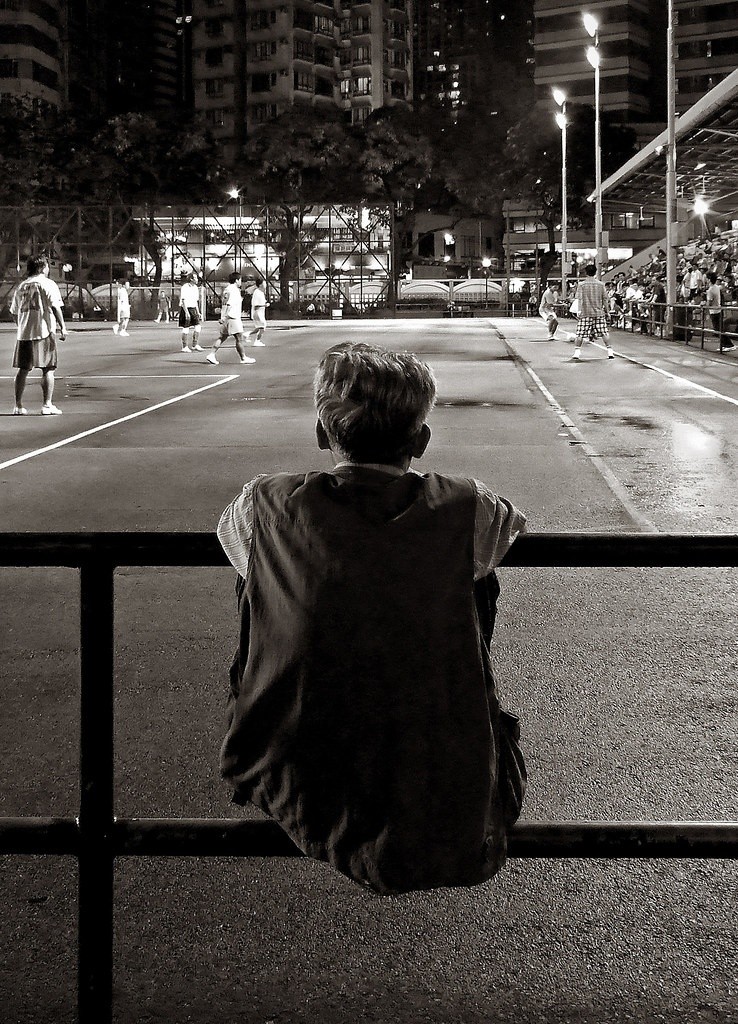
[243,332,251,342]
[120,330,129,336]
[192,344,204,350]
[113,325,118,335]
[13,407,27,414]
[181,346,192,352]
[717,345,736,352]
[206,353,220,364]
[241,356,256,364]
[41,403,62,414]
[253,340,265,346]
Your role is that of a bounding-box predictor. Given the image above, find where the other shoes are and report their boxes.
[548,338,554,341]
[610,320,693,342]
[154,320,160,323]
[608,351,614,359]
[572,354,580,360]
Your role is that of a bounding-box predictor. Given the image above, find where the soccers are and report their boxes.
[568,333,576,342]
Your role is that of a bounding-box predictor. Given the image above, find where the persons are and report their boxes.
[539,282,560,340]
[207,272,256,364]
[603,224,738,354]
[154,291,171,323]
[178,272,205,352]
[216,341,528,896]
[244,279,270,346]
[570,265,614,359]
[529,295,537,316]
[9,256,68,414]
[113,280,131,336]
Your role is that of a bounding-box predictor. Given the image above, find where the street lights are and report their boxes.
[228,188,243,277]
[580,11,604,282]
[551,87,571,297]
